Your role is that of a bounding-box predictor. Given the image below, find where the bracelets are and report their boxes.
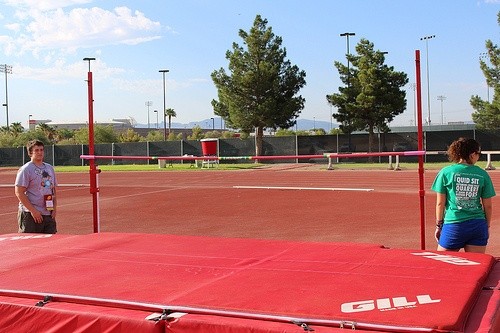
[436,220,443,228]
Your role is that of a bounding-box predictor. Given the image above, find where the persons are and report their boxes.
[14,141,57,234]
[430,137,495,252]
